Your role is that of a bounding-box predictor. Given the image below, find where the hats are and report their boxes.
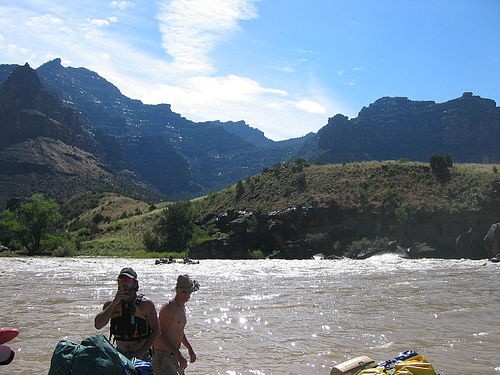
[117,267,137,280]
[175,274,194,291]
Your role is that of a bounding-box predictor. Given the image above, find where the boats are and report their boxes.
[155,257,200,265]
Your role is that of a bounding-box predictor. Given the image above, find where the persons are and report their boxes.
[496,254,500,258]
[152,275,201,375]
[169,255,173,261]
[95,268,161,375]
[160,255,164,260]
[183,254,189,261]
